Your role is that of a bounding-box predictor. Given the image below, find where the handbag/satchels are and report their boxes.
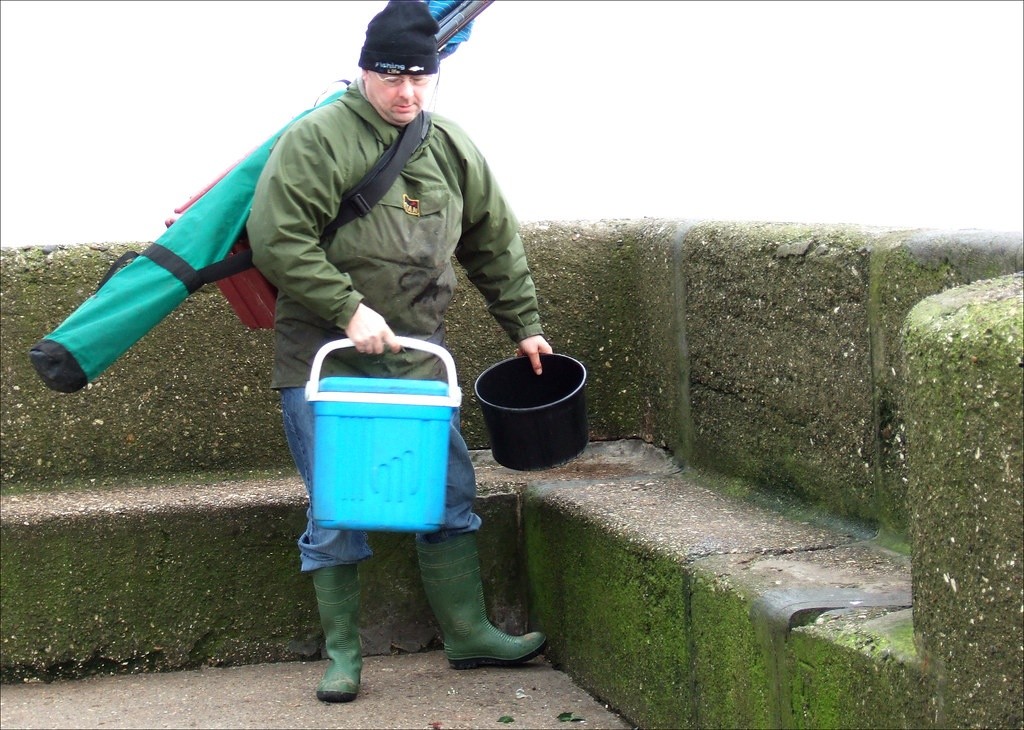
[28,0,475,395]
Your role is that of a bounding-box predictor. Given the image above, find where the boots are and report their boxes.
[308,563,363,704]
[413,532,548,670]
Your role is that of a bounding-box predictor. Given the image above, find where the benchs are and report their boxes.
[1,213,1024,730]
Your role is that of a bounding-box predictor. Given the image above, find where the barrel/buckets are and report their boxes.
[303,336,461,533]
[474,354,588,471]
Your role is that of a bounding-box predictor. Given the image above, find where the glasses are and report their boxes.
[374,71,435,86]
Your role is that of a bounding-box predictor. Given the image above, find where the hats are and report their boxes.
[358,1,441,74]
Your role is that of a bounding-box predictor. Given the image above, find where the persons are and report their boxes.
[247,0,557,704]
